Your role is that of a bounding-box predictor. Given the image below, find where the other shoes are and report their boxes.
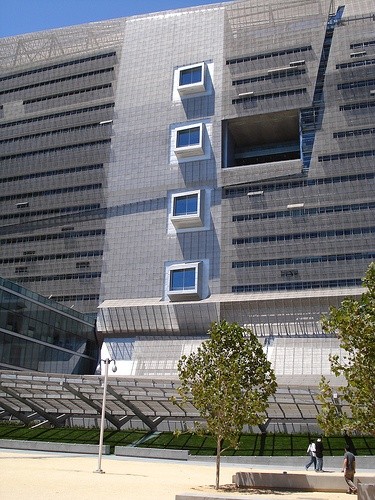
[346,491,351,494]
[315,469,324,473]
[354,489,357,494]
[304,466,308,471]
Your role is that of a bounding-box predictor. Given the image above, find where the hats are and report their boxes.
[317,438,321,442]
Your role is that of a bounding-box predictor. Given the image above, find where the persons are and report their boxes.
[342,445,357,494]
[314,436,324,472]
[305,439,317,470]
